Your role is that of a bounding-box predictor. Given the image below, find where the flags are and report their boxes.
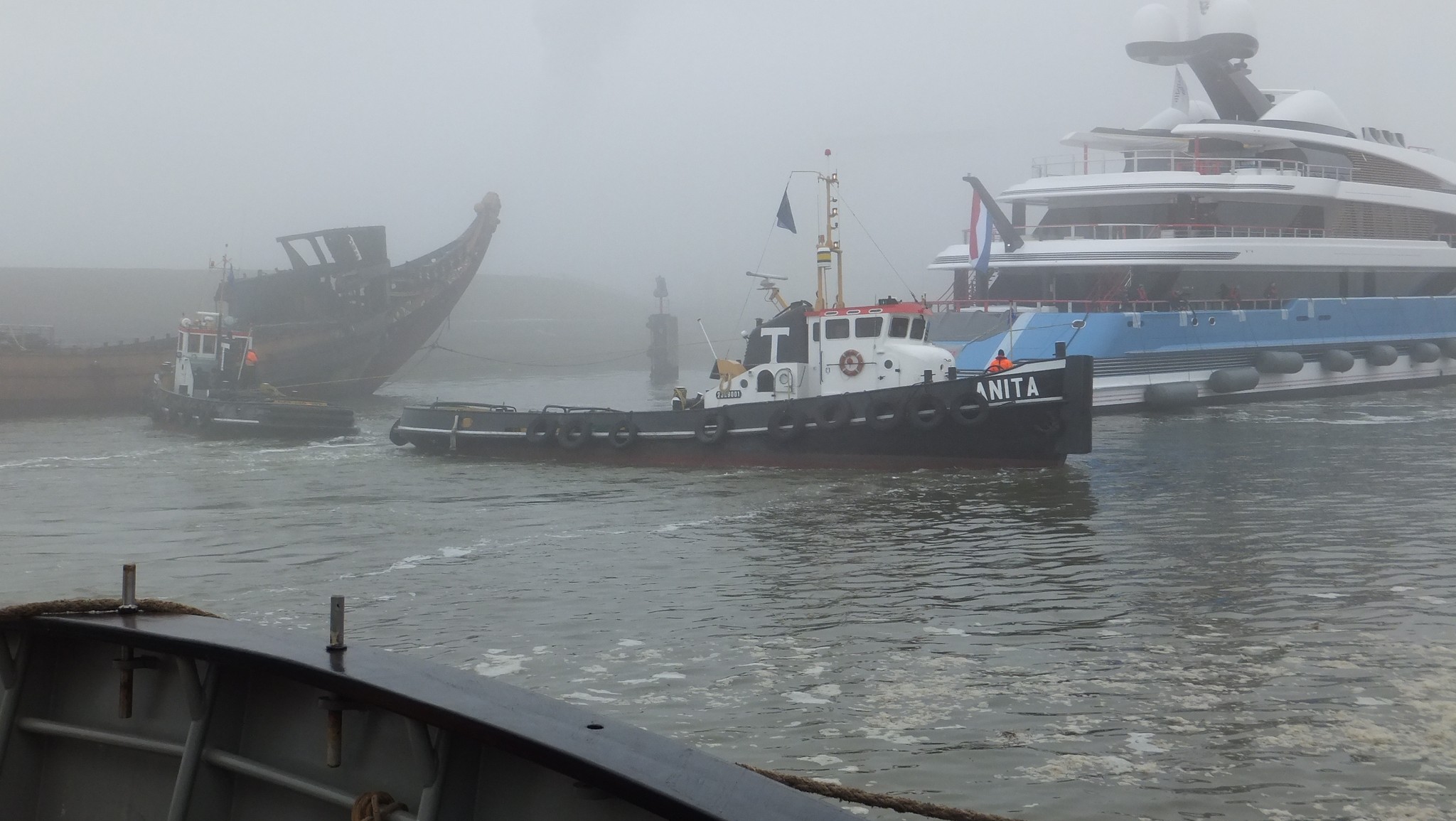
[968,189,993,274]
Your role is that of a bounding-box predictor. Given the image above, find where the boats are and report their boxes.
[648,275,682,382]
[144,244,359,440]
[387,149,1095,470]
[1,197,501,417]
[921,30,1456,416]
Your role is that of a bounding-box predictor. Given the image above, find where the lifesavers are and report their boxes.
[839,350,864,376]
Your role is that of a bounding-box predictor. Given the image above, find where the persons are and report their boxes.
[237,351,258,385]
[982,274,1270,310]
[989,349,1013,374]
